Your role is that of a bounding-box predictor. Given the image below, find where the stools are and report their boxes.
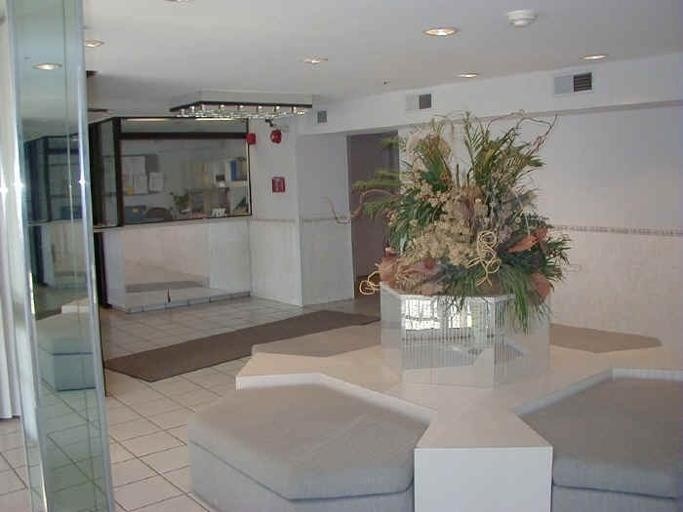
[520,375,683,512]
[188,382,425,512]
[548,322,662,353]
[251,324,378,357]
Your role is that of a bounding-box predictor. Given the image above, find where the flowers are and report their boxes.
[325,108,572,335]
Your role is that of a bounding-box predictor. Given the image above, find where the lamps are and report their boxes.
[169,93,312,124]
